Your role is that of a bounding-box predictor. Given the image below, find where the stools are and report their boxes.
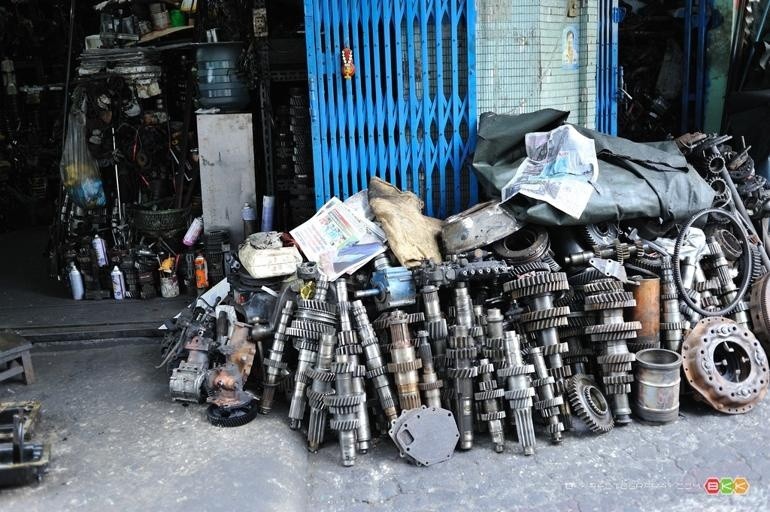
[1,332,36,386]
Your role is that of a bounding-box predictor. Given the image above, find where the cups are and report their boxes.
[159,268,180,298]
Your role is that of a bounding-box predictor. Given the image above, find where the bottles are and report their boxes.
[1,57,17,94]
[182,215,204,246]
[240,202,256,220]
[92,234,108,267]
[111,266,126,300]
[194,253,209,289]
[68,266,85,301]
